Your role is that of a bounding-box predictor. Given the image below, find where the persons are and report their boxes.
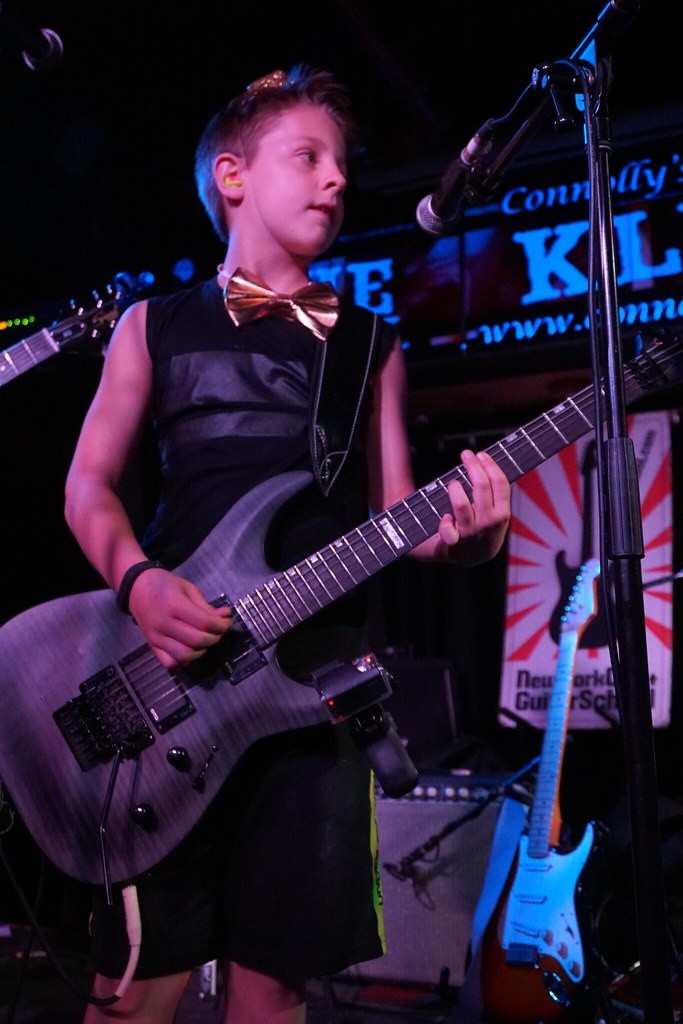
[62,68,511,1024]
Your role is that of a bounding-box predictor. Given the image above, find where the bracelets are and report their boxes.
[117,560,161,616]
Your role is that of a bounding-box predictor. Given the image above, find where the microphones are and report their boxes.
[416,118,499,237]
[9,22,63,73]
[383,862,427,883]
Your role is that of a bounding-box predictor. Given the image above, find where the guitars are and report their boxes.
[1,325,682,888]
[469,551,595,1022]
[0,267,162,388]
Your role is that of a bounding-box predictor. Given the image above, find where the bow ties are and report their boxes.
[216,263,339,342]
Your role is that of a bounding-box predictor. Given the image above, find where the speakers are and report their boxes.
[332,770,534,989]
